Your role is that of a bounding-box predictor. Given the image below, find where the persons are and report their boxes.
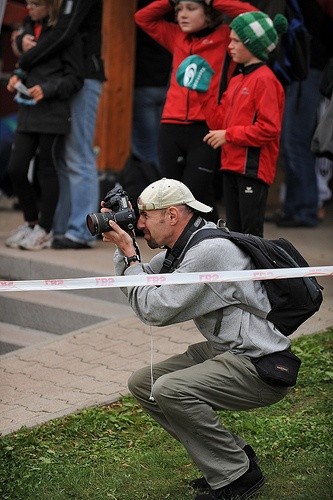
[133,0,261,228]
[132,0,179,181]
[101,177,303,500]
[0,0,108,250]
[176,11,286,238]
[250,0,333,228]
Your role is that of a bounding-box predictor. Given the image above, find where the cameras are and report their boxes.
[86,189,137,236]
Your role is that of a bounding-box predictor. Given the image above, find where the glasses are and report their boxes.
[26,0,45,8]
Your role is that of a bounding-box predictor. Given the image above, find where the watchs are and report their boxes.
[123,255,141,266]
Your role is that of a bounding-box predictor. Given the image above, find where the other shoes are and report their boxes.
[191,446,266,500]
[277,211,319,227]
[4,223,55,251]
[52,234,90,248]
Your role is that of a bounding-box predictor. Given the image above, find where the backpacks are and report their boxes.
[179,227,324,337]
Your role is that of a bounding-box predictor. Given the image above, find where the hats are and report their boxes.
[229,12,288,62]
[137,176,213,214]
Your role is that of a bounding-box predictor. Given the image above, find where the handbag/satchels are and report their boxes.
[253,351,302,387]
